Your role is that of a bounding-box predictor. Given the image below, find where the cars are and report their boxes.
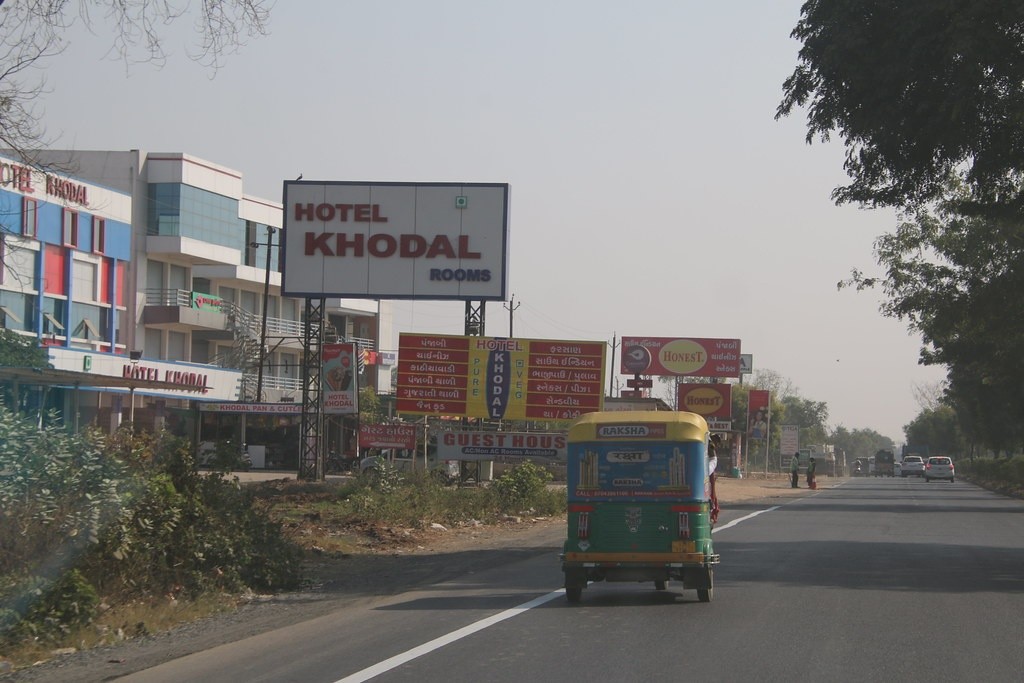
[867,455,931,478]
[922,456,955,483]
[900,455,926,477]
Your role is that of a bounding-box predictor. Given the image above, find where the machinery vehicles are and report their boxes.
[849,457,869,477]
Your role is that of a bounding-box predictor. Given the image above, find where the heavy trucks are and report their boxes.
[349,430,567,484]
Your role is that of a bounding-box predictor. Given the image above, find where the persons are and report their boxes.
[750,410,766,438]
[806,458,816,489]
[709,432,721,518]
[790,452,799,489]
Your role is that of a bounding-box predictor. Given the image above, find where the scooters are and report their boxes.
[227,441,253,469]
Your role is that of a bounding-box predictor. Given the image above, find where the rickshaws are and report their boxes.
[874,450,896,478]
[560,410,721,602]
